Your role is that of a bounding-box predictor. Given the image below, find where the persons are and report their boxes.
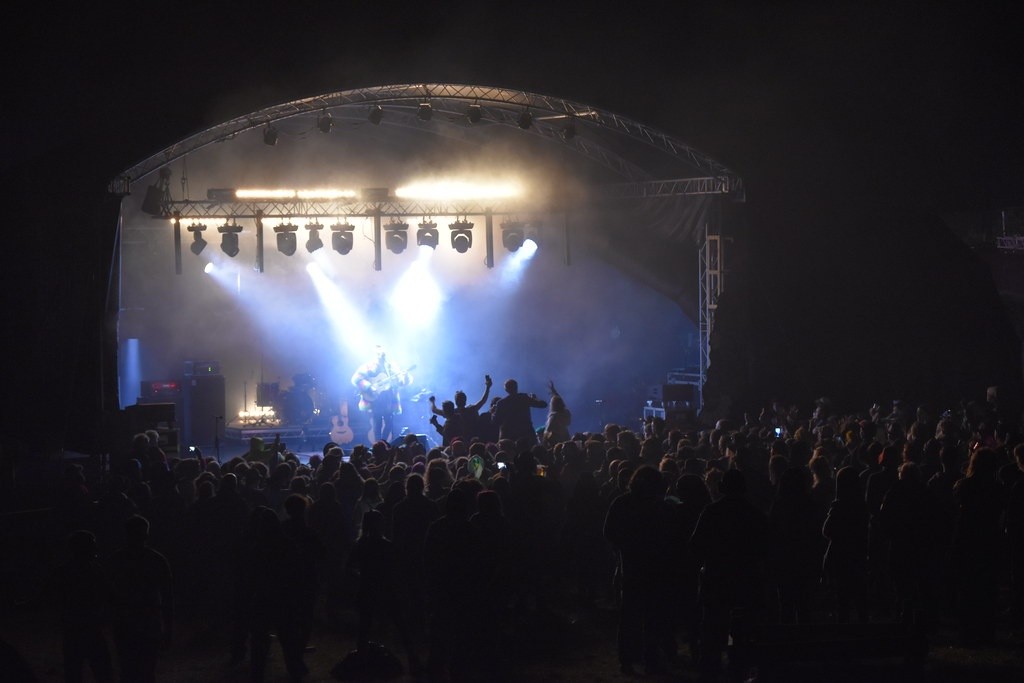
[45,368,1023,683]
[351,346,413,444]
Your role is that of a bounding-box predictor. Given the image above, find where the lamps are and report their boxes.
[465,101,481,124]
[516,106,533,130]
[316,112,334,134]
[330,224,355,255]
[367,104,384,126]
[186,225,207,255]
[273,225,298,256]
[417,223,439,255]
[448,222,474,253]
[562,119,576,139]
[305,224,324,253]
[416,102,433,121]
[141,184,163,215]
[263,126,279,146]
[383,223,409,254]
[217,226,243,257]
[499,221,524,252]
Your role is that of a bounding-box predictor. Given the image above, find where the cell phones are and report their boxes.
[775,428,781,438]
[497,463,505,469]
[189,447,195,452]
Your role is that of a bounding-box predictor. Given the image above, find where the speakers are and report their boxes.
[126,374,225,459]
[697,290,746,427]
[398,434,437,455]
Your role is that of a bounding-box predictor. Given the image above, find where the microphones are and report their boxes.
[215,416,223,419]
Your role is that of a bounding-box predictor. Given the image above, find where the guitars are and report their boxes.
[330,396,353,445]
[360,363,416,402]
[367,407,392,444]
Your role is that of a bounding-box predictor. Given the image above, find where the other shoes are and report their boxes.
[287,660,310,675]
[688,646,699,667]
[409,655,426,675]
[231,646,247,665]
[248,664,264,683]
[619,663,633,674]
[640,660,668,674]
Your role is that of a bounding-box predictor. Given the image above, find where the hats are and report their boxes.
[919,403,931,415]
[816,396,832,407]
[370,441,386,452]
